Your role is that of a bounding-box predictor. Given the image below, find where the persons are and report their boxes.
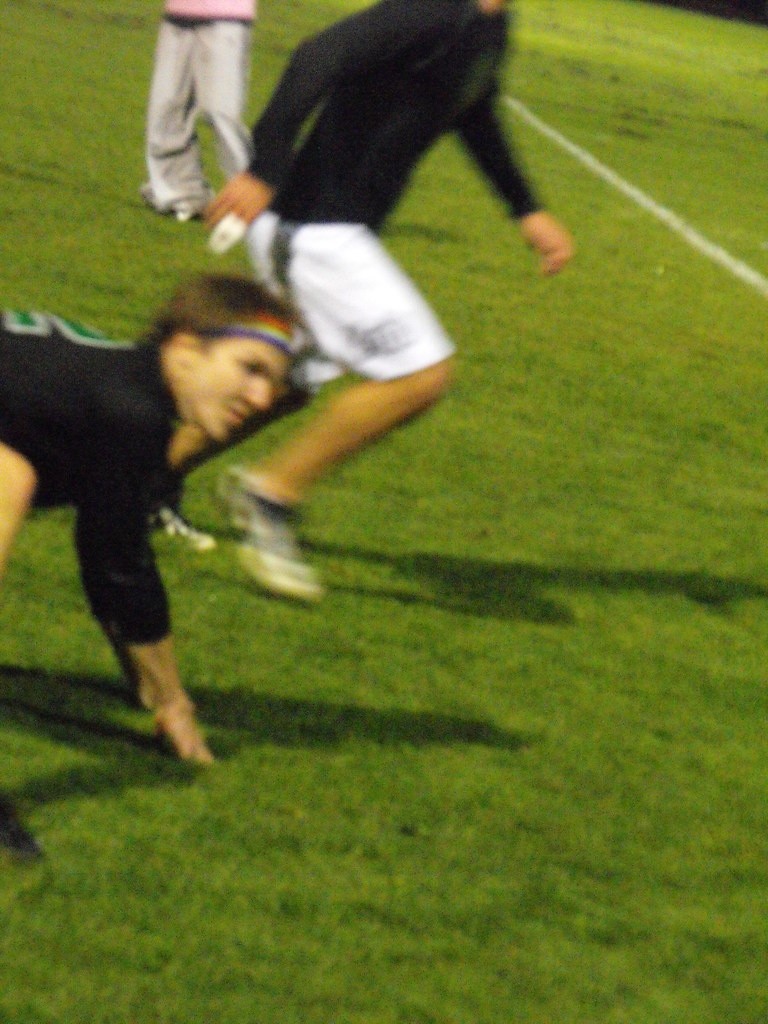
[140,0,258,222]
[147,0,578,606]
[0,270,302,864]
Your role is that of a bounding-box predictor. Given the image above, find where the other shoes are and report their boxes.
[139,182,215,219]
[151,497,219,554]
[214,467,328,604]
